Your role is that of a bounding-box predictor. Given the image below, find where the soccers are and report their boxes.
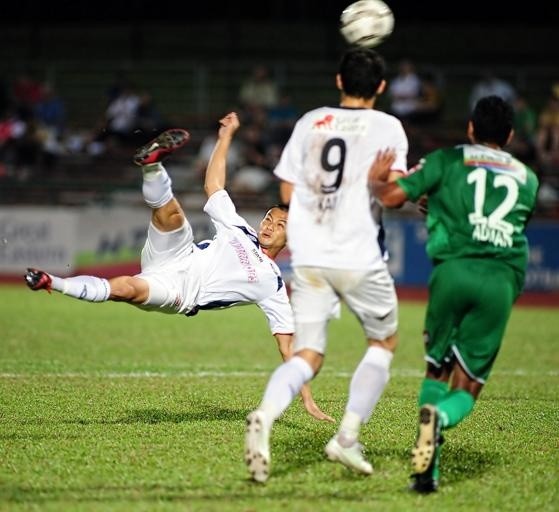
[341,0,395,48]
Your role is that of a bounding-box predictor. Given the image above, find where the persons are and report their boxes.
[369,95,543,494]
[192,63,306,191]
[23,107,334,423]
[244,46,411,485]
[381,61,559,216]
[0,62,175,206]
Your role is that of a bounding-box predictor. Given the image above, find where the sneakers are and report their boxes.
[410,405,442,495]
[24,268,52,292]
[322,435,374,475]
[244,412,270,482]
[133,129,190,167]
